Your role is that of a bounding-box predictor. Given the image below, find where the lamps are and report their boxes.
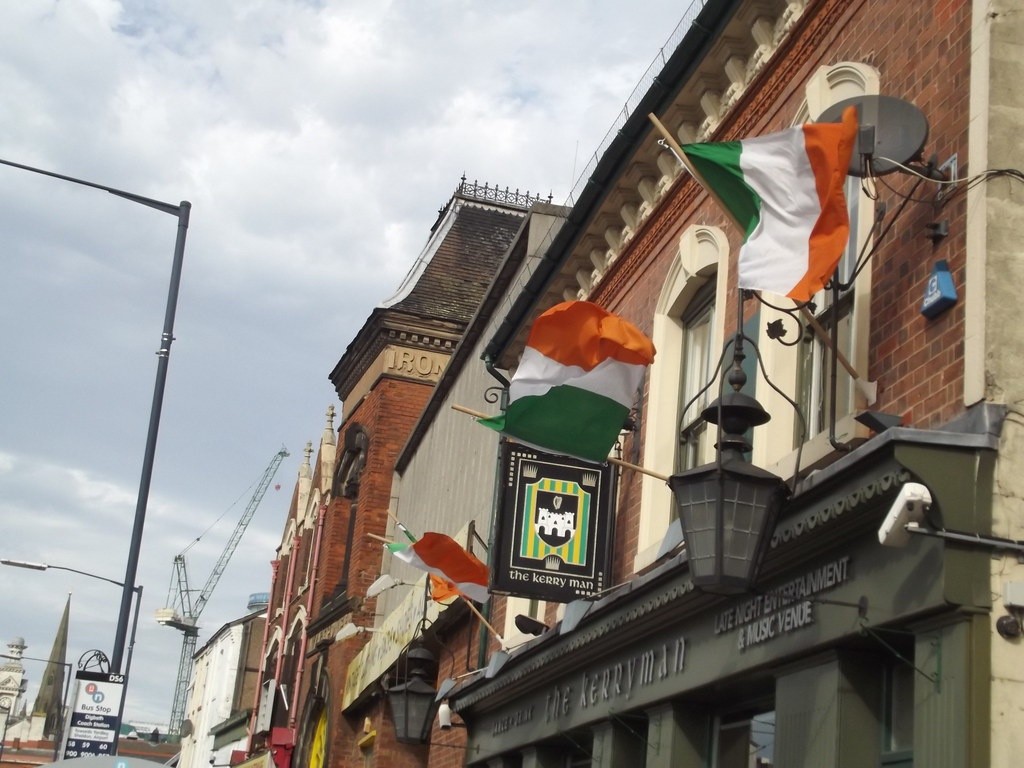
[364,574,416,598]
[558,580,632,634]
[853,409,903,433]
[484,640,528,679]
[665,286,867,619]
[515,614,550,636]
[382,573,481,755]
[435,667,484,701]
[336,623,379,642]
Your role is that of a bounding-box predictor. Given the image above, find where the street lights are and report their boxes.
[0,653,73,764]
[0,557,143,756]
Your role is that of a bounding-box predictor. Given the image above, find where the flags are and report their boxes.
[681,105,858,301]
[385,532,493,603]
[476,299,656,464]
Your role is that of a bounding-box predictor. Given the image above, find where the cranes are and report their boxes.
[154,441,291,745]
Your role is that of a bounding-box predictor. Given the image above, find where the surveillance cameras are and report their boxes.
[877,484,933,548]
[438,703,452,731]
